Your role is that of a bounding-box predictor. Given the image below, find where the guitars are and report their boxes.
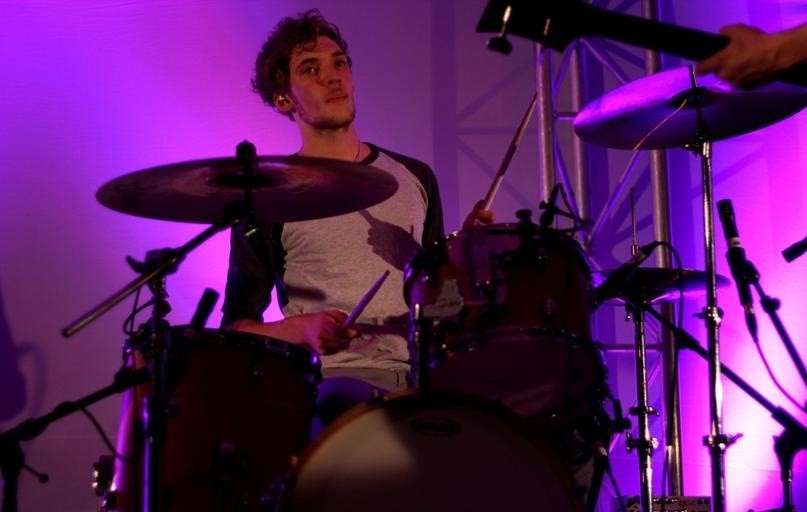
[471,1,807,87]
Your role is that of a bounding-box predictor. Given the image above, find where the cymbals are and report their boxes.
[573,65,806,149]
[96,154,400,225]
[591,271,726,296]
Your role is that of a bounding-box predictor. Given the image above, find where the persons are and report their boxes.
[694,22,807,91]
[219,7,498,392]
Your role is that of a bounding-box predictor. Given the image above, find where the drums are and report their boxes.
[617,496,712,510]
[406,226,607,418]
[106,326,321,510]
[293,389,579,510]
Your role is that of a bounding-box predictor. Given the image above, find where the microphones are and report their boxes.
[581,240,657,315]
[717,199,757,336]
